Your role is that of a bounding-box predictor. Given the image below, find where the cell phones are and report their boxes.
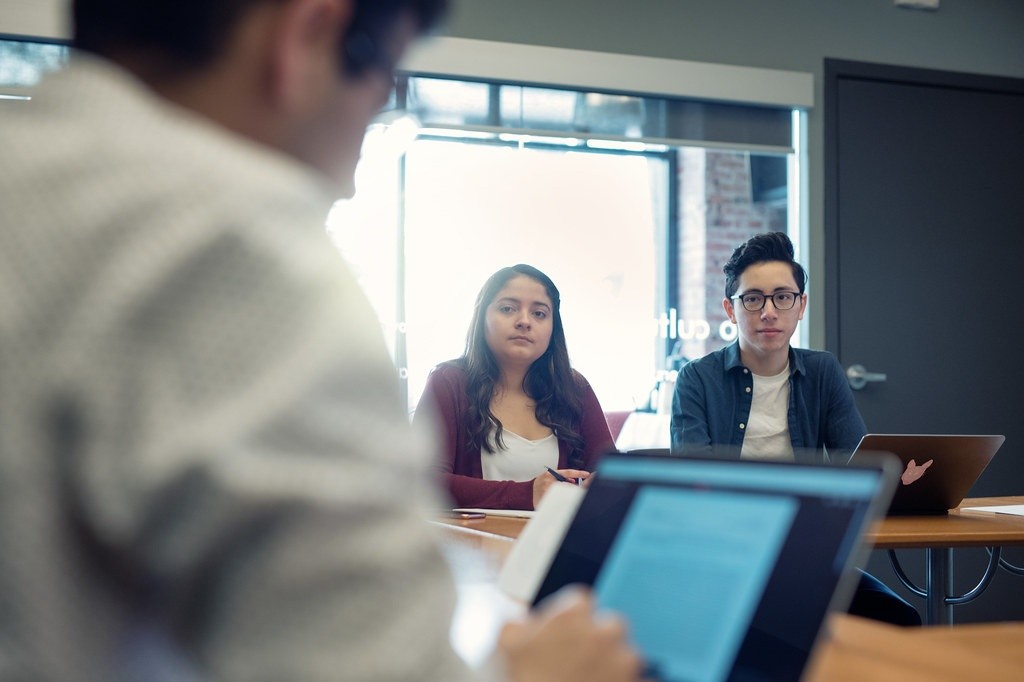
[446,511,486,519]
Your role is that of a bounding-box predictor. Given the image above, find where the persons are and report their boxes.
[3,0,642,682]
[670,233,921,629]
[412,265,620,509]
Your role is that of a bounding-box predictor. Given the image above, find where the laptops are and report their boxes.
[845,434,1005,516]
[530,447,905,682]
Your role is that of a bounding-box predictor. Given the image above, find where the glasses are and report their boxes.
[730,290,802,312]
[361,25,423,167]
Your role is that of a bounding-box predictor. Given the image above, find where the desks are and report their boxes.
[428,496,1024,682]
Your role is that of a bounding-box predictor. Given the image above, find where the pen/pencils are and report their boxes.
[545,464,568,482]
